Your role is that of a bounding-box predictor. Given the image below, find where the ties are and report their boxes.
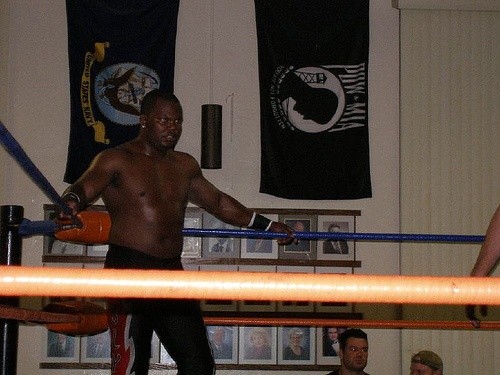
[333,242,341,254]
[219,246,224,252]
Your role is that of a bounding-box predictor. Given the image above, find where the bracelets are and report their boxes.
[60,192,80,203]
[248,211,273,231]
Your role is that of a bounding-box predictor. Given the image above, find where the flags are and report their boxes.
[253,0,373,200]
[63,0,180,184]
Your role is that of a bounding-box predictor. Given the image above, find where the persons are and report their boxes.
[47,333,73,357]
[410,350,443,375]
[87,333,110,358]
[465,205,500,327]
[55,88,298,375]
[211,226,233,252]
[327,328,371,375]
[286,220,310,252]
[283,327,310,361]
[209,327,233,359]
[323,326,339,356]
[324,224,349,254]
[244,326,271,360]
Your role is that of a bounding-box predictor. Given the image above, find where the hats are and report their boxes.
[411,350,443,374]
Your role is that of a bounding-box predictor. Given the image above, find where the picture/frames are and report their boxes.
[38,204,361,371]
